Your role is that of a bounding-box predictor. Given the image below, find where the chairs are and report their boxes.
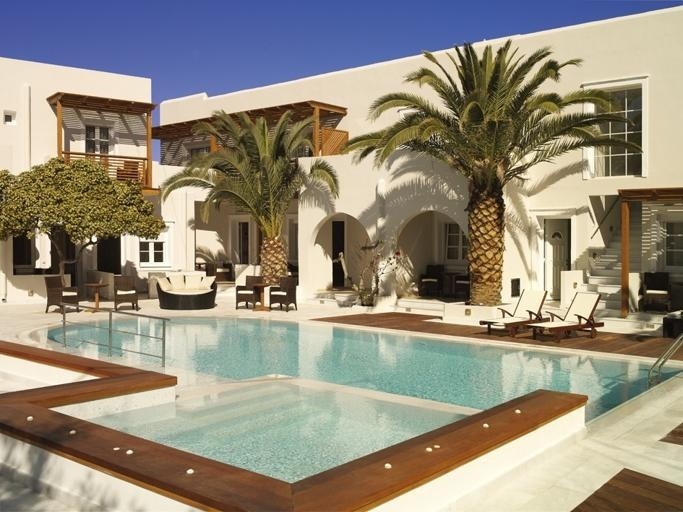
[112,275,139,312]
[640,272,672,309]
[417,262,445,296]
[454,273,470,300]
[480,290,601,340]
[43,275,81,316]
[235,274,298,312]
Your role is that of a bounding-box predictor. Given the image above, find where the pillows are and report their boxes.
[156,273,216,293]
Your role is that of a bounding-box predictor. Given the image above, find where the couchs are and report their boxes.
[154,271,219,309]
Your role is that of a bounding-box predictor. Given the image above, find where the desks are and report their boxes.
[82,283,110,313]
[443,272,459,299]
